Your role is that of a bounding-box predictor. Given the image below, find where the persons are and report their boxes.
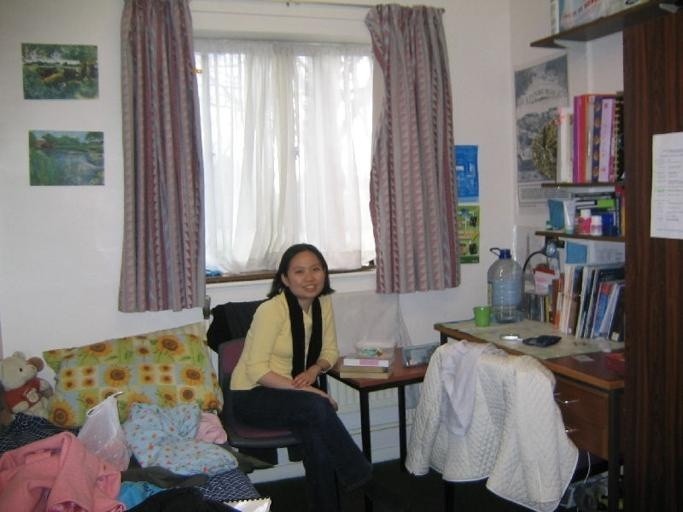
[229,243,383,512]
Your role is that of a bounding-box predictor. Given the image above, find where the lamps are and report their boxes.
[516,240,557,312]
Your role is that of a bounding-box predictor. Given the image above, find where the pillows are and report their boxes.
[40,316,225,431]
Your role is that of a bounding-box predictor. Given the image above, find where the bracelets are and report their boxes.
[314,362,325,372]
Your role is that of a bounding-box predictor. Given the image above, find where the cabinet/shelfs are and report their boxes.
[531,181,626,243]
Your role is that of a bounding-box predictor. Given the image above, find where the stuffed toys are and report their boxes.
[27,357,53,398]
[0,351,47,419]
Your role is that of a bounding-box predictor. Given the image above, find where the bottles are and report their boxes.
[576,208,591,233]
[589,216,602,238]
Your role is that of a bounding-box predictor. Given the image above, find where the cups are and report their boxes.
[473,306,491,326]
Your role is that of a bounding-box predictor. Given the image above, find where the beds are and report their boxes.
[0,407,272,512]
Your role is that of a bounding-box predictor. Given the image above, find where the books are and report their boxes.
[545,93,623,341]
[339,366,389,374]
[339,358,395,368]
[338,374,394,379]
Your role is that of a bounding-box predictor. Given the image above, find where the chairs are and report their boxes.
[405,338,581,512]
[206,298,333,469]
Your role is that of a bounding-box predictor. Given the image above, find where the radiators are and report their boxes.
[326,288,406,416]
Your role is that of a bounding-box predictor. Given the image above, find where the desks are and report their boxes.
[434,313,624,506]
[326,339,441,470]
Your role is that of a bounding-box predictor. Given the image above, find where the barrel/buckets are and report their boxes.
[487,247,523,323]
